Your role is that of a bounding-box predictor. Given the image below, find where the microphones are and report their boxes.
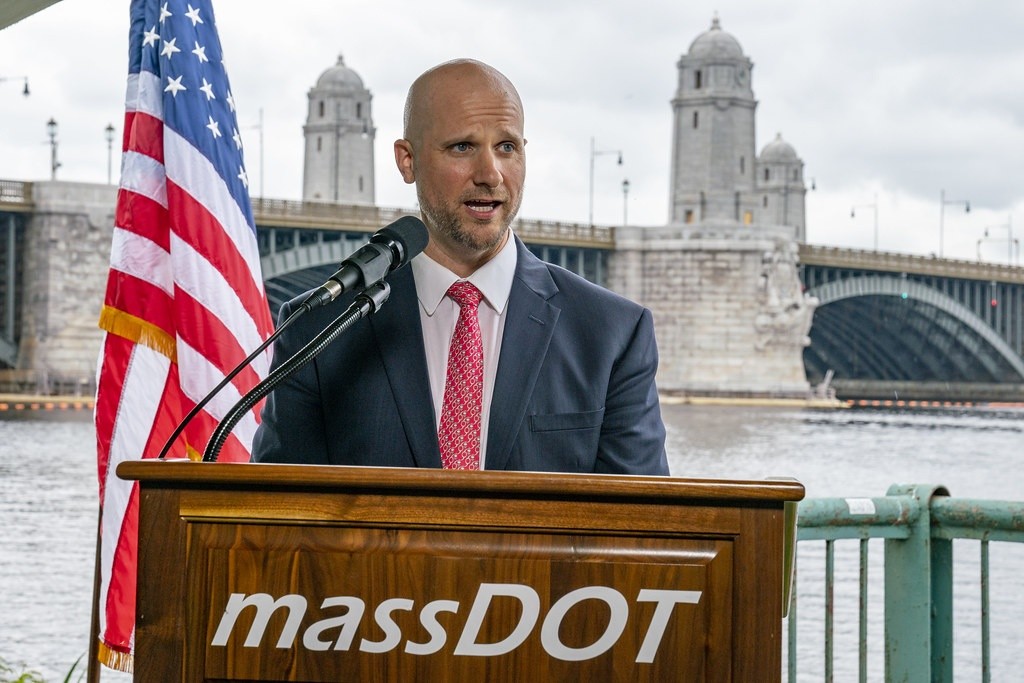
[301,215,430,312]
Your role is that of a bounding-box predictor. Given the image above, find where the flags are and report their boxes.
[92,0,274,674]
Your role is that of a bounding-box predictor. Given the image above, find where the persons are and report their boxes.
[248,56,670,476]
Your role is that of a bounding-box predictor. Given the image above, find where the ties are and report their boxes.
[438,281,485,471]
